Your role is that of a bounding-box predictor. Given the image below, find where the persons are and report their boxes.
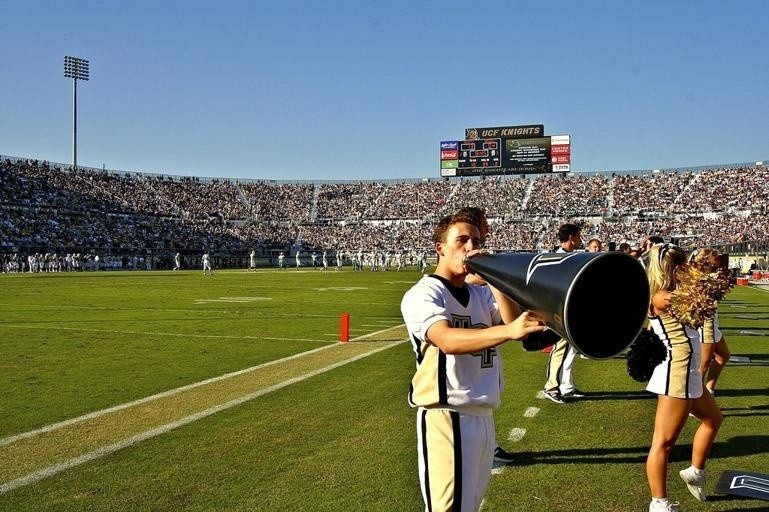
[645,245,723,512]
[400,213,546,511]
[459,206,518,463]
[544,224,585,405]
[687,245,730,398]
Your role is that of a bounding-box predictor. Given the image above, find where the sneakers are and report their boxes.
[493,447,515,463]
[649,501,676,512]
[542,388,586,404]
[678,466,707,502]
